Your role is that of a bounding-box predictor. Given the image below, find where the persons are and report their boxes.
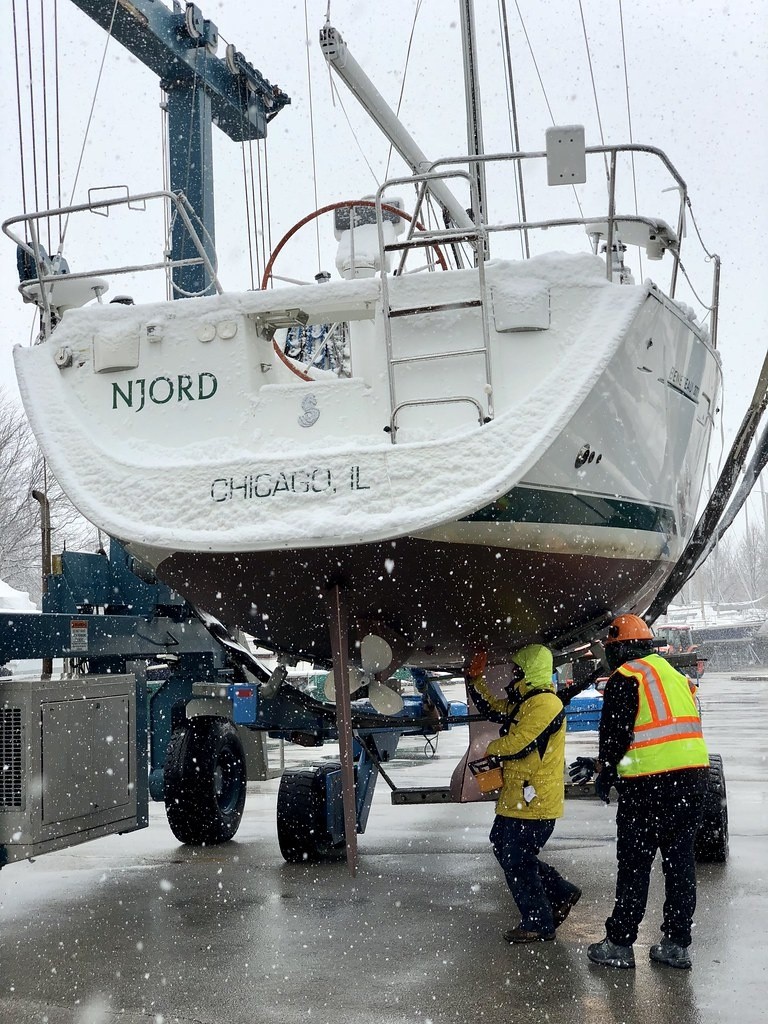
[466,642,582,944]
[568,613,708,968]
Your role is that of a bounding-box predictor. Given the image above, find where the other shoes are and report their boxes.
[554,885,580,929]
[504,924,554,942]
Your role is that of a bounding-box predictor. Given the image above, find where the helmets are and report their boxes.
[604,615,654,644]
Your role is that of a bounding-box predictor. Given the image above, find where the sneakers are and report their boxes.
[649,939,691,969]
[587,937,634,968]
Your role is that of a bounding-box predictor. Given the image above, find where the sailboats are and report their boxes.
[0,0,726,682]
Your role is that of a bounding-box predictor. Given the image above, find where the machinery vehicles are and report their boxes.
[652,625,705,679]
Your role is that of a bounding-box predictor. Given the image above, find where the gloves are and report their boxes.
[568,757,596,786]
[594,767,619,803]
[469,647,487,676]
